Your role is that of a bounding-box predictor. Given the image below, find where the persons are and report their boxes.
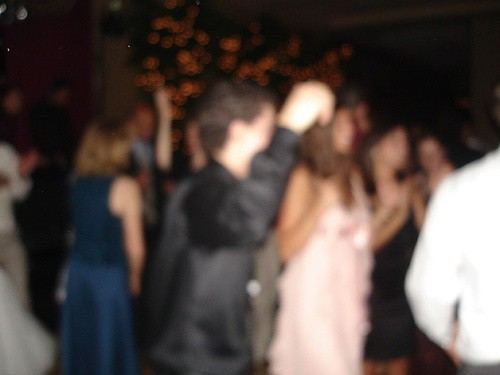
[0,70,500,375]
